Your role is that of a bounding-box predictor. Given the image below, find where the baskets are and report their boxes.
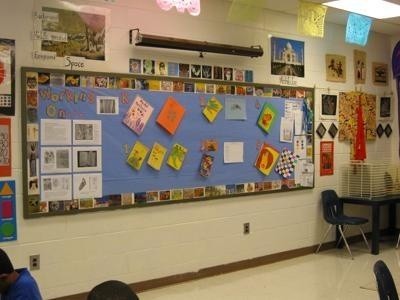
[345,159,400,200]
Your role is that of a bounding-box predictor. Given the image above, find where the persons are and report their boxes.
[84,280,140,299]
[0,246,43,300]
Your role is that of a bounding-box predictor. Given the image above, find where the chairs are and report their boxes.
[315,190,369,260]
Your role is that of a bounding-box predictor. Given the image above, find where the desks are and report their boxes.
[336,193,400,254]
